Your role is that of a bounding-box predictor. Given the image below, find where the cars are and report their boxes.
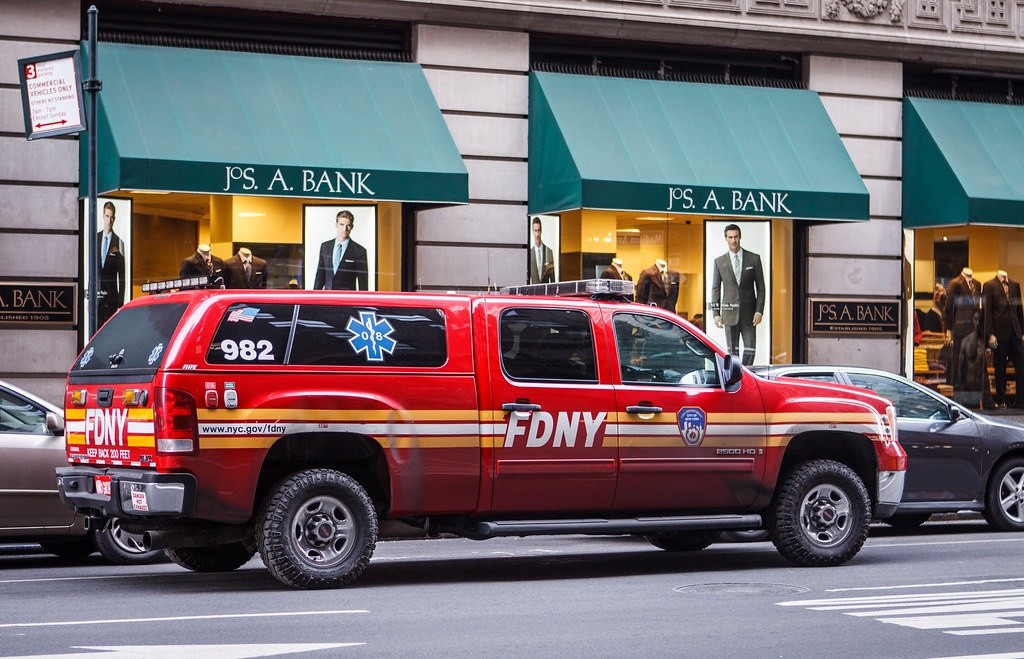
[1,382,169,565]
[748,364,1024,534]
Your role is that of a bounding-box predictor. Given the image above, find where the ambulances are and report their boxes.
[53,278,909,594]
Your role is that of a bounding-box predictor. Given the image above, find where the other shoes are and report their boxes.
[999,399,1007,410]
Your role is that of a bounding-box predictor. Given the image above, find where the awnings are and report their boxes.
[901,95,1024,228]
[76,40,470,213]
[527,69,870,225]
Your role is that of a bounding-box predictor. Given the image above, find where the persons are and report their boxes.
[710,224,766,366]
[528,216,556,286]
[97,201,125,333]
[594,258,680,367]
[943,267,1024,409]
[313,210,368,291]
[513,328,552,364]
[179,242,269,289]
[957,309,983,394]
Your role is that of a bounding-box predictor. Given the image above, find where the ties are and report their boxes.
[334,244,342,275]
[207,259,213,276]
[735,255,741,286]
[969,281,975,306]
[621,271,625,280]
[662,272,668,297]
[246,260,251,283]
[537,249,541,272]
[102,236,108,271]
